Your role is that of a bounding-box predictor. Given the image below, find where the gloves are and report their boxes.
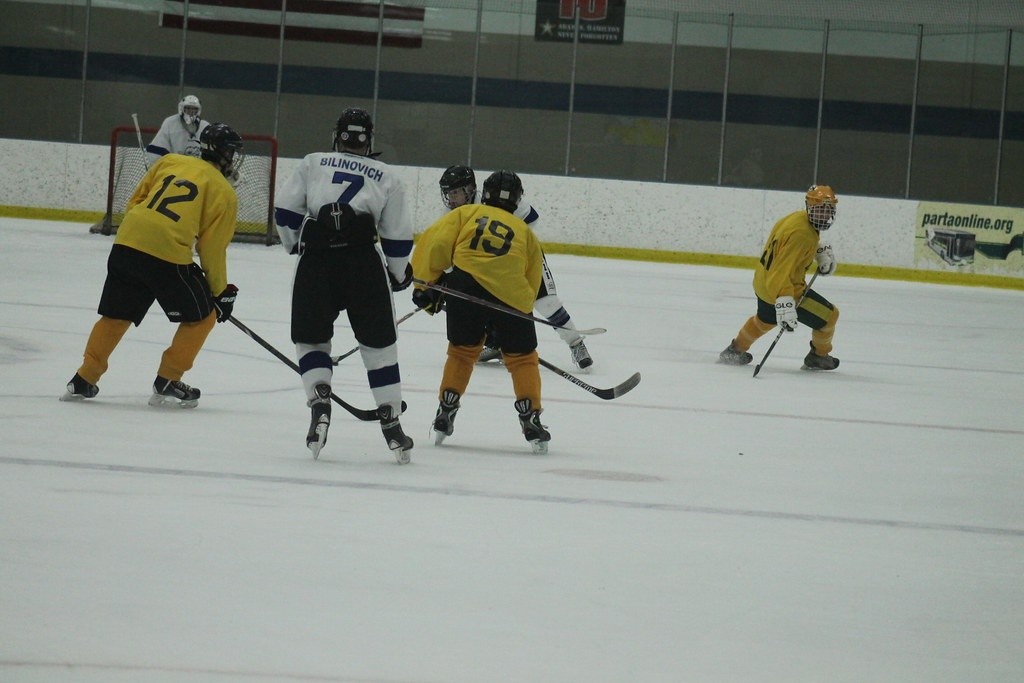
[775,296,799,333]
[815,242,836,277]
[386,262,414,292]
[213,284,239,323]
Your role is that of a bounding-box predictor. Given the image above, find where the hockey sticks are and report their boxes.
[412,277,607,335]
[228,314,407,422]
[131,113,151,171]
[331,308,422,363]
[441,305,642,400]
[752,265,820,377]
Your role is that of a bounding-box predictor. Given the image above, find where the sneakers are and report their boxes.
[718,338,753,366]
[475,346,504,364]
[514,398,551,454]
[429,389,460,446]
[800,340,841,373]
[59,372,99,401]
[378,405,414,465]
[306,384,331,460]
[148,375,201,409]
[569,340,594,375]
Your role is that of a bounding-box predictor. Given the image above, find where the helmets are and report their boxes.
[481,171,524,214]
[439,165,477,210]
[200,122,246,181]
[332,108,376,154]
[178,95,202,125]
[805,185,838,231]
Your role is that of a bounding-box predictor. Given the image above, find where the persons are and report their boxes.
[146,95,241,190]
[274,107,414,465]
[409,168,551,455]
[59,123,244,411]
[716,185,840,371]
[438,164,594,374]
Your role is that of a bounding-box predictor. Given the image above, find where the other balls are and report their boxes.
[332,362,338,366]
